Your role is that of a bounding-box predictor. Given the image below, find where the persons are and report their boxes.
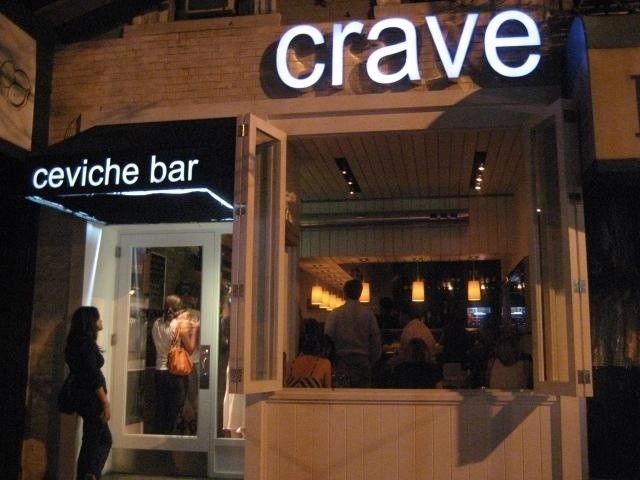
[59,306,114,480]
[283,271,535,395]
[137,279,247,478]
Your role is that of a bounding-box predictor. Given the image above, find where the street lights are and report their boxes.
[166,320,194,378]
[55,374,84,414]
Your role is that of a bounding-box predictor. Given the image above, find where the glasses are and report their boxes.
[308,269,344,312]
[411,260,425,302]
[356,264,371,302]
[466,260,482,302]
